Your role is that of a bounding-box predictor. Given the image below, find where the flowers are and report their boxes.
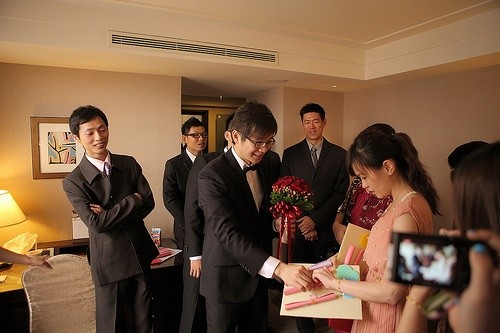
[270,176,318,264]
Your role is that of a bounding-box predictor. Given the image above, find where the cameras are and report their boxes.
[390,234,486,291]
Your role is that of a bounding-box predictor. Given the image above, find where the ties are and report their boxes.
[312,146,318,170]
[104,162,110,180]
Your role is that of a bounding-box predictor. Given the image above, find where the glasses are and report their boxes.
[186,131,208,138]
[242,133,276,148]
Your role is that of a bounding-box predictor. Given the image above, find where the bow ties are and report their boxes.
[244,165,257,173]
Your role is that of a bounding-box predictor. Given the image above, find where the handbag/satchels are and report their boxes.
[343,213,351,226]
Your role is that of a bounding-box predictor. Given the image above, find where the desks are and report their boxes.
[0,259,34,294]
[148,236,184,271]
[30,238,89,256]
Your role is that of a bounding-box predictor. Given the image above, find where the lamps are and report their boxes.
[0,189,28,229]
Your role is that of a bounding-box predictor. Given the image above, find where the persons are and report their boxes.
[62,105,160,333]
[0,246,52,269]
[163,99,322,333]
[282,103,351,333]
[312,123,444,333]
[396,141,500,333]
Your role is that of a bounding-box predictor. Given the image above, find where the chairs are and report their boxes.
[21,253,98,333]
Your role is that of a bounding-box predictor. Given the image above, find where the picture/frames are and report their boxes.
[30,116,86,178]
